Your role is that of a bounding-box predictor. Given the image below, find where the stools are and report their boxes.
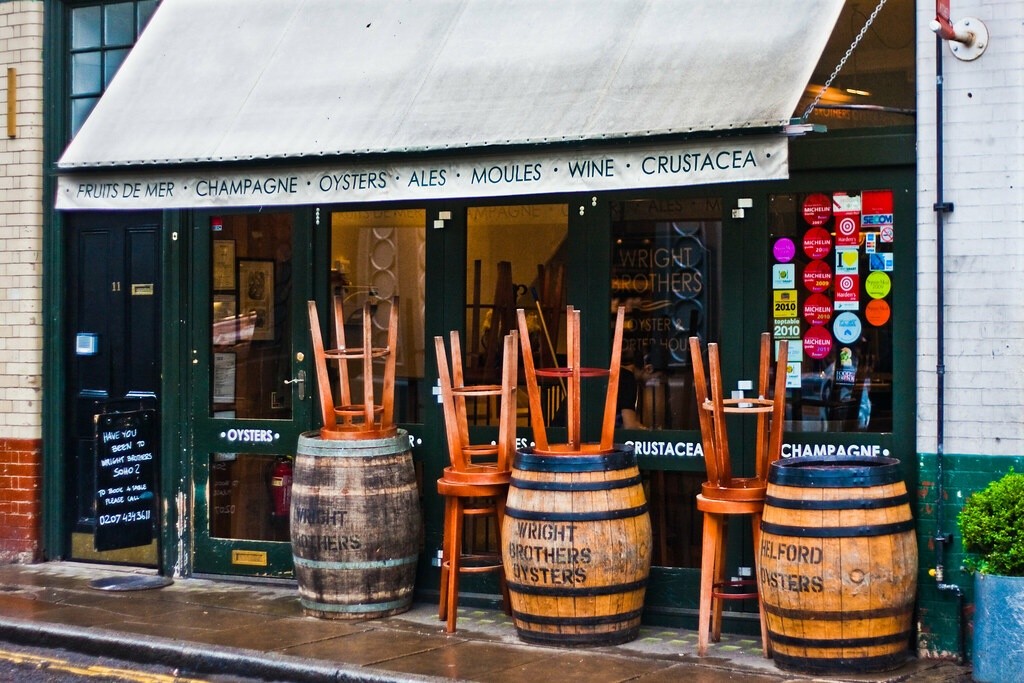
[433,304,627,634]
[689,332,790,659]
[464,256,563,382]
[308,294,401,441]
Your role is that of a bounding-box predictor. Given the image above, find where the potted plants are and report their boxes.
[956,465,1024,683]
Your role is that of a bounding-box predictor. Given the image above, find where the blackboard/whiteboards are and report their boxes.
[93,408,160,552]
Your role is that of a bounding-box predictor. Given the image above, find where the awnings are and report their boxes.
[52,0,888,209]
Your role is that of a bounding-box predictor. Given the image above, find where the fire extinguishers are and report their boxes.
[270,454,294,518]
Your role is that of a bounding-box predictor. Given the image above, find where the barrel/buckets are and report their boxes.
[289,429,420,620]
[502,444,652,647]
[759,456,919,674]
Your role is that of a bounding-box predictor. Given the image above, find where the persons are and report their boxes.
[818,338,872,430]
[553,342,670,430]
